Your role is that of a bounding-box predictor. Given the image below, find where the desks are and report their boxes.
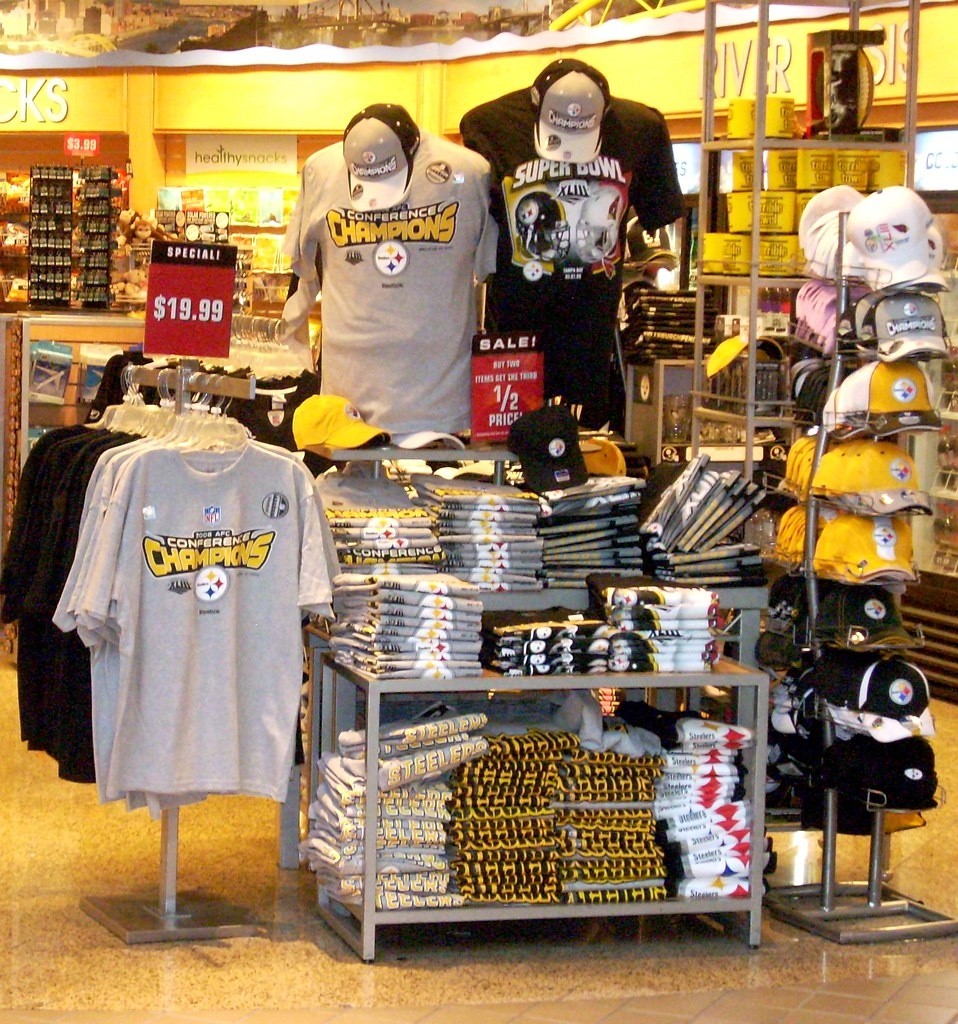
[312,653,771,965]
[279,586,769,870]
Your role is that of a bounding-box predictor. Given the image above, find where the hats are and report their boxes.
[795,281,949,362]
[786,435,934,516]
[770,667,815,739]
[581,440,625,477]
[801,185,951,293]
[343,105,419,212]
[707,333,786,378]
[813,648,936,742]
[846,733,937,809]
[508,404,589,492]
[767,573,917,652]
[292,394,390,449]
[391,430,466,450]
[623,277,657,295]
[776,504,917,584]
[623,216,677,266]
[792,359,942,439]
[529,59,609,163]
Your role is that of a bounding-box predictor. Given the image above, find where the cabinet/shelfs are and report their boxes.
[910,283,958,579]
[690,0,921,545]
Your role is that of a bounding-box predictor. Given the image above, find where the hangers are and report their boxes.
[84,364,249,453]
